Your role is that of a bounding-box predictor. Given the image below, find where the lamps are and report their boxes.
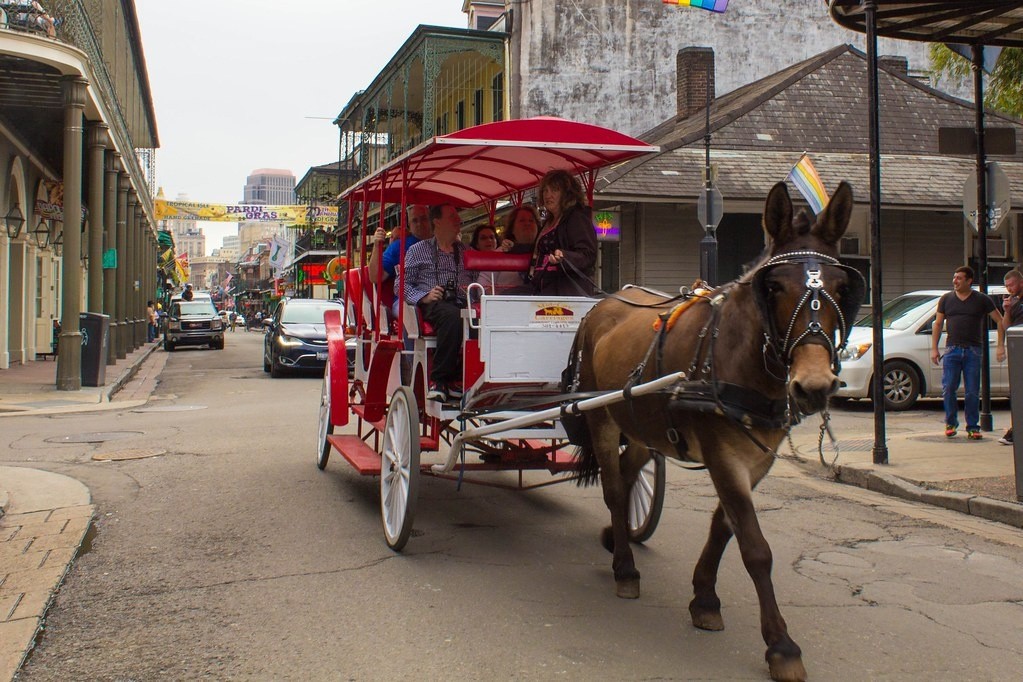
[4,203,25,239]
[54,231,64,257]
[83,254,88,270]
[34,218,51,248]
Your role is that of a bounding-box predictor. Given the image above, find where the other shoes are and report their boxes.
[151,339,156,343]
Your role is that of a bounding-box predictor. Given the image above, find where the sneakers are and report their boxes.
[425,382,447,402]
[449,382,463,397]
[968,429,982,440]
[998,428,1013,445]
[945,423,959,438]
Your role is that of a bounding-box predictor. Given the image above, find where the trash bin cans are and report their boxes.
[1006,323,1023,502]
[79,312,110,386]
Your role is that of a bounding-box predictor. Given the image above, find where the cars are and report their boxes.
[218,310,245,327]
[830,286,1010,411]
[159,301,227,350]
[169,293,212,306]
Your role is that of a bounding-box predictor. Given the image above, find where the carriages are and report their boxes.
[317,117,868,681]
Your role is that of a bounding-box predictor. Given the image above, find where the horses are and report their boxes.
[566,181,867,682]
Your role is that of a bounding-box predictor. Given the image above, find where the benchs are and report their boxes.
[341,250,534,421]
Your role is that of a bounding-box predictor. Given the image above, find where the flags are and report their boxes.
[157,246,192,283]
[268,233,308,270]
[788,154,830,216]
[221,271,233,287]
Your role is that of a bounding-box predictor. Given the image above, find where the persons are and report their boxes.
[998,265,1023,446]
[242,308,272,332]
[229,309,237,332]
[147,300,164,343]
[181,284,193,301]
[930,266,1006,440]
[367,168,597,402]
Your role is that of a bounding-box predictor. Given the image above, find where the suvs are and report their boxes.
[262,296,357,379]
